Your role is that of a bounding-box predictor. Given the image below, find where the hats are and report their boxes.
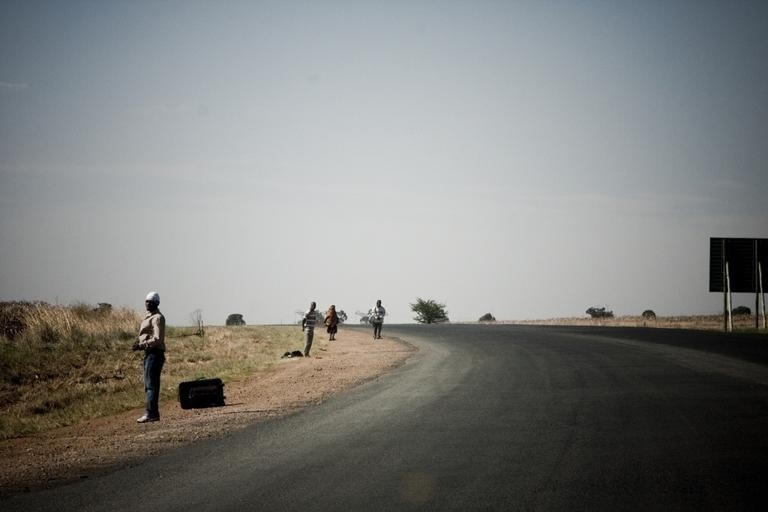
[145,292,160,301]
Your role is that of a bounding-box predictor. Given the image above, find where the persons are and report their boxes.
[369,299,385,339]
[300,301,316,358]
[130,292,166,423]
[322,305,339,342]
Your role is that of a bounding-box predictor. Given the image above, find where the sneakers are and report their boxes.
[136,414,160,423]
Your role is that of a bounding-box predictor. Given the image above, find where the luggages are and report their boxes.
[178,378,226,410]
